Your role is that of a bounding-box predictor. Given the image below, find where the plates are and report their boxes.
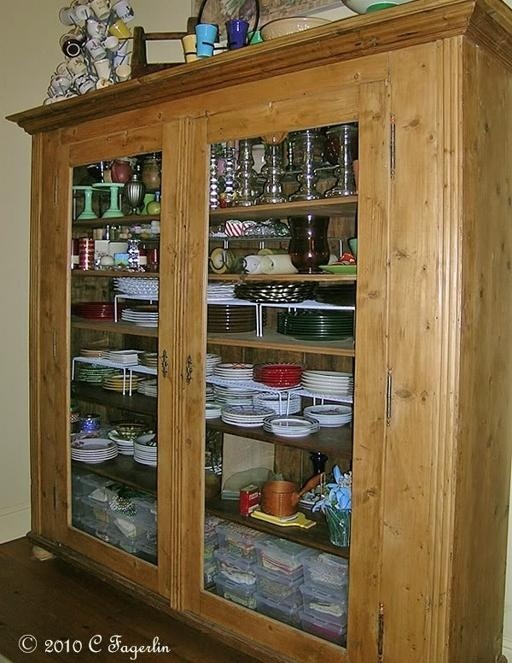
[318,265,357,273]
[70,277,358,468]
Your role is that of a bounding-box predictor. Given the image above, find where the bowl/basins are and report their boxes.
[343,0,417,14]
[260,16,333,41]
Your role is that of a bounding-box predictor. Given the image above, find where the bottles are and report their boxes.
[286,218,329,274]
[309,454,329,477]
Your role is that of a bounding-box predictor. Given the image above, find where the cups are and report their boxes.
[182,19,264,64]
[42,0,137,106]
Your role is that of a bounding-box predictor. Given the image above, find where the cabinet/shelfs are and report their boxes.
[6,6,506,662]
